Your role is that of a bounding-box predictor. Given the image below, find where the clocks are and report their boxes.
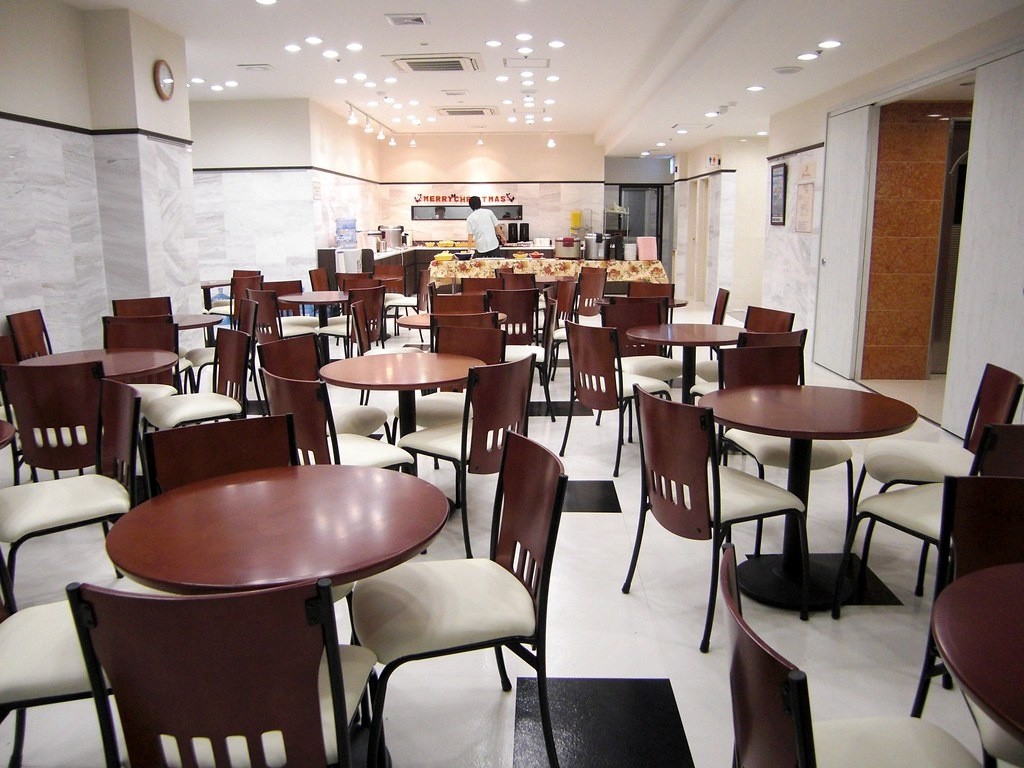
[155,59,175,100]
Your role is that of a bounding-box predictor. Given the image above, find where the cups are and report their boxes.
[614,234,624,261]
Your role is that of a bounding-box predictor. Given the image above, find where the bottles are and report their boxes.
[335,217,357,249]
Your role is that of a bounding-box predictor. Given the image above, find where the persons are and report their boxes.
[435,207,446,219]
[467,196,507,259]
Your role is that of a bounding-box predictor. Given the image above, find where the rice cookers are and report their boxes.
[554,237,581,259]
[585,233,611,260]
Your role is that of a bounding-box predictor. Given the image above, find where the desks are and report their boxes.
[318,352,486,479]
[592,295,688,308]
[396,311,507,329]
[533,275,576,305]
[105,464,449,604]
[169,313,223,332]
[0,249,1024,768]
[928,558,1023,749]
[625,323,746,405]
[17,346,178,380]
[696,384,918,612]
[277,290,350,365]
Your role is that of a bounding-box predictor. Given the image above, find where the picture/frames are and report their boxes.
[770,162,786,225]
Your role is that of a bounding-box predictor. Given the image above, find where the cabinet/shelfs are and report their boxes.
[403,250,417,296]
[508,251,555,260]
[416,250,456,291]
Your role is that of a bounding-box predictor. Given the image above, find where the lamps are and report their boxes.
[344,99,567,149]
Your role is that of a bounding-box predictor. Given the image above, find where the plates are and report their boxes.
[529,253,543,258]
[455,253,471,260]
[513,253,528,259]
[434,254,455,260]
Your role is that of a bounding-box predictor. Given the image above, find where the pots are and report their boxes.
[376,225,404,249]
